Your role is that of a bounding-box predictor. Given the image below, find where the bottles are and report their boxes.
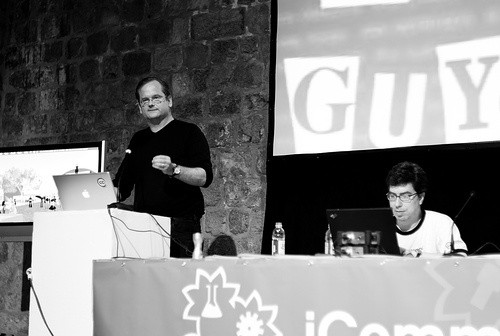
[325,224,335,255]
[272,222,285,256]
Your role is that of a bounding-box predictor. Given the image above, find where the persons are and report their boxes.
[112,77,213,258]
[386,163,468,258]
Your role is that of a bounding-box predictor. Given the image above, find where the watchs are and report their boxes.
[172,164,180,178]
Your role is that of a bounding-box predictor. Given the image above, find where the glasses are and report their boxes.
[384,189,421,204]
[136,95,167,107]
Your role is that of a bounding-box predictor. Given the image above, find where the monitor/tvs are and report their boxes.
[0,140,106,242]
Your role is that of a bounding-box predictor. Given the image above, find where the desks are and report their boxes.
[93,254,500,335]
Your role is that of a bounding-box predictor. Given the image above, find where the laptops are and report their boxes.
[53,172,117,210]
[326,209,403,256]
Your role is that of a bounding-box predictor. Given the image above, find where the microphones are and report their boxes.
[108,149,132,212]
[443,193,474,257]
